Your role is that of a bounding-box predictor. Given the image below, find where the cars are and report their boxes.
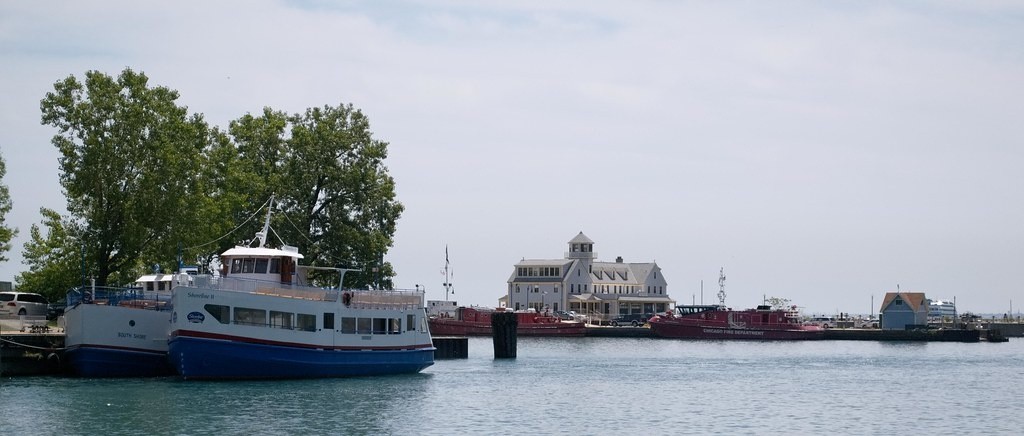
[551,310,573,321]
[859,319,880,328]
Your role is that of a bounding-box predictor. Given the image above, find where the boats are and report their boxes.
[61,266,200,380]
[166,190,439,386]
[649,305,828,339]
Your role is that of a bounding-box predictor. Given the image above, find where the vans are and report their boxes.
[809,316,837,329]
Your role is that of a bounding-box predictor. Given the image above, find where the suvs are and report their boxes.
[611,313,648,327]
[0,292,53,317]
